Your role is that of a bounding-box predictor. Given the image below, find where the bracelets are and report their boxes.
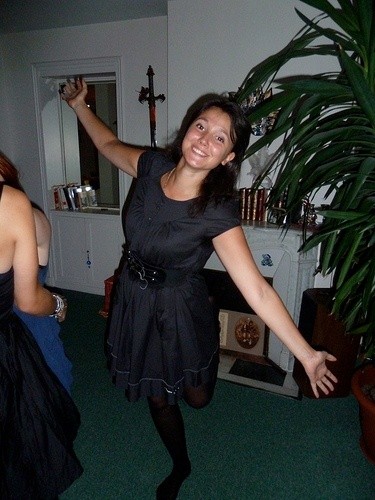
[73,104,92,114]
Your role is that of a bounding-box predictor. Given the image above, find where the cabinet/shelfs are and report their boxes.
[46,215,126,297]
[195,225,320,374]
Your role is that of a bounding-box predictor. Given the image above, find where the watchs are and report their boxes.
[49,294,65,319]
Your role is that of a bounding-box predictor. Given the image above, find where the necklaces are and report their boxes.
[163,167,176,193]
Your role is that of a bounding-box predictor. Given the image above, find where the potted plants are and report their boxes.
[232,0,375,464]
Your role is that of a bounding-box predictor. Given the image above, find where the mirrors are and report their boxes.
[29,58,125,212]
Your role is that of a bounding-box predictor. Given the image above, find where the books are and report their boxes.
[54,183,98,211]
[239,188,266,221]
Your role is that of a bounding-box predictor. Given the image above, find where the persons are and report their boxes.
[61,80,338,500]
[0,151,84,500]
[14,201,74,397]
[241,319,258,346]
